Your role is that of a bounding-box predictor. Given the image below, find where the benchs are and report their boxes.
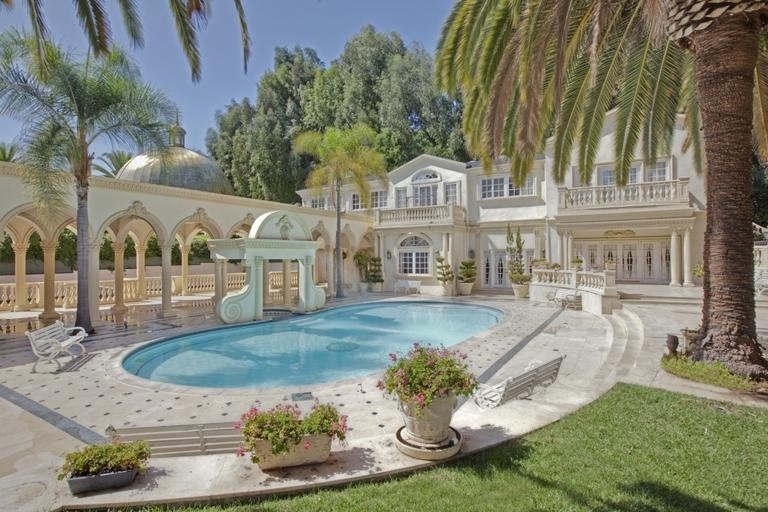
[23,317,90,374]
[546,285,580,311]
[393,279,422,297]
[468,352,569,411]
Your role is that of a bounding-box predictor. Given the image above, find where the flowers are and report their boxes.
[232,397,353,463]
[376,339,482,421]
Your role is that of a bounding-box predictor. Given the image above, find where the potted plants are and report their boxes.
[352,249,386,295]
[433,248,479,296]
[58,435,153,494]
[505,221,533,298]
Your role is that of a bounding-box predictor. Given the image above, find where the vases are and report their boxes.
[391,391,464,461]
[250,434,333,470]
